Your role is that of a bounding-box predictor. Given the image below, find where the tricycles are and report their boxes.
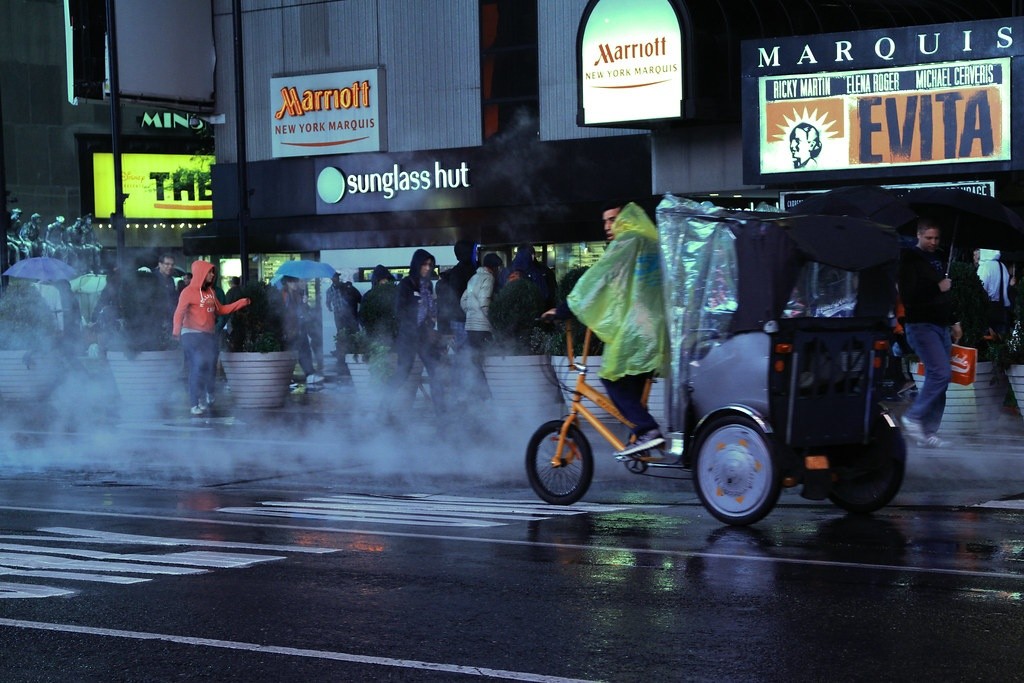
[523,190,907,526]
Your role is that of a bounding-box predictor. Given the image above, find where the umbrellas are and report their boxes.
[895,186,1024,238]
[276,259,339,282]
[787,187,920,231]
[0,253,79,283]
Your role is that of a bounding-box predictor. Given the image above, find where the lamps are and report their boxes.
[189,113,225,125]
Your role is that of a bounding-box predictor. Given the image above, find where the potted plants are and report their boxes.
[907,259,1004,433]
[1,271,69,402]
[539,266,622,417]
[988,260,1024,416]
[333,282,426,412]
[101,276,189,406]
[221,280,300,408]
[479,279,565,408]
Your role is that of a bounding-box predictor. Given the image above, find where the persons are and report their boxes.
[542,201,667,457]
[857,218,1024,448]
[0,244,555,415]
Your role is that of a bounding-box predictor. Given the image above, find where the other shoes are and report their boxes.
[191,406,202,415]
[306,374,325,383]
[197,403,207,411]
[613,428,665,458]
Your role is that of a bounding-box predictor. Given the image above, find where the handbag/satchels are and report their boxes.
[915,344,978,388]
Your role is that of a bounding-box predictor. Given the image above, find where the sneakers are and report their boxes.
[901,416,928,445]
[917,434,954,449]
